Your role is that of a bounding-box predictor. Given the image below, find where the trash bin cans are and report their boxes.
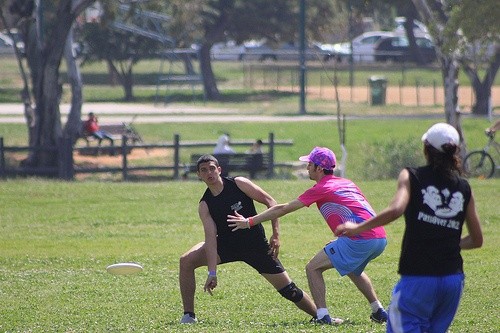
[368,76,389,105]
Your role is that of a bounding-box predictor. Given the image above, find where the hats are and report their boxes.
[422,123,460,155]
[299,146,336,171]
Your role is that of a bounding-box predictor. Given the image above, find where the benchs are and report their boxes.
[181,153,271,179]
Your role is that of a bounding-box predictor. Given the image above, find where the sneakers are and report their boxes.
[309,313,332,326]
[370,308,388,323]
[330,318,345,326]
[180,314,199,324]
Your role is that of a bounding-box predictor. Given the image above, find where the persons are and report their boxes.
[333,123,485,333]
[246,140,264,155]
[227,147,389,324]
[179,154,343,324]
[487,120,500,135]
[84,112,114,146]
[214,136,233,155]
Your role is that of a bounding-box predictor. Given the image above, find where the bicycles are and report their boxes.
[463,129,500,180]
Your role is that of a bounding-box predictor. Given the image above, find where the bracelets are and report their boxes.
[247,217,255,229]
[209,271,216,276]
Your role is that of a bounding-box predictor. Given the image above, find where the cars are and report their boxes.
[189,16,500,66]
[0,30,25,54]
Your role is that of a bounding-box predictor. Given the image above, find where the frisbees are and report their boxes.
[107,263,143,274]
